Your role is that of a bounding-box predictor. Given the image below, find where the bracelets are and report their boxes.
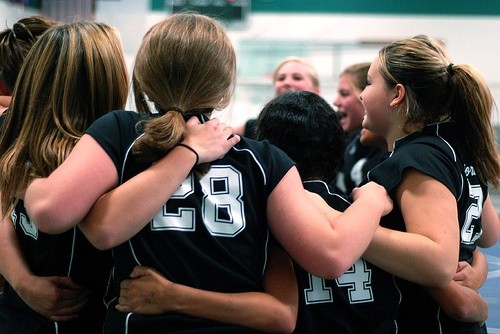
[176,142,200,168]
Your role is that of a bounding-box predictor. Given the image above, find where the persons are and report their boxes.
[0,15,92,324]
[333,62,380,195]
[1,21,245,334]
[232,54,319,143]
[114,91,489,334]
[17,11,394,334]
[301,34,499,334]
[358,126,392,177]
